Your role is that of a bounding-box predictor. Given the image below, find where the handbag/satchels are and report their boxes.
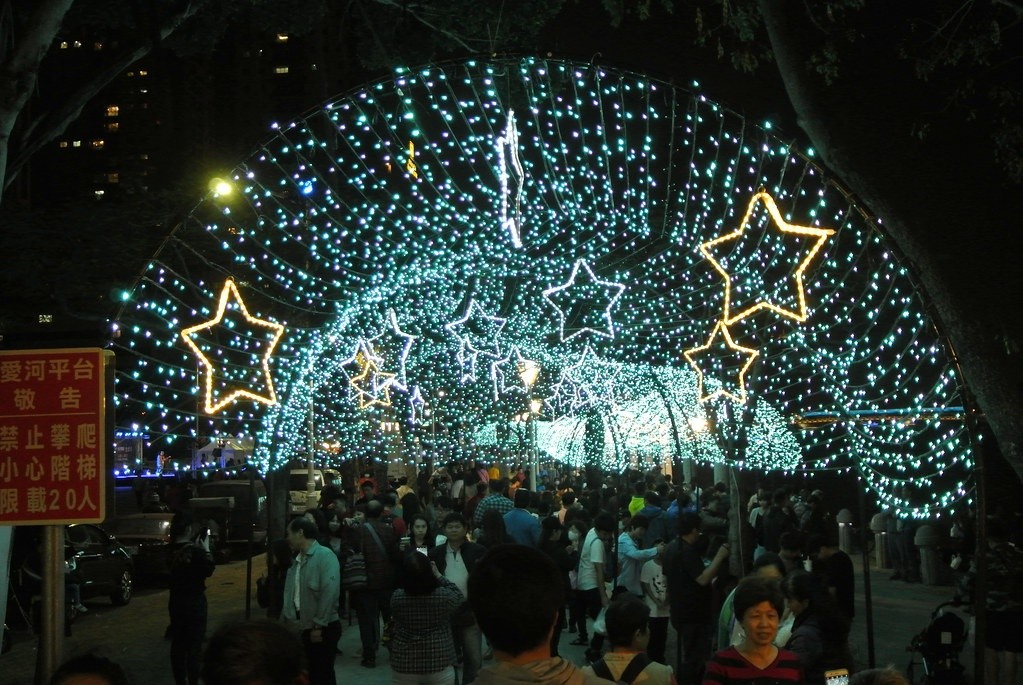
[344,576,381,614]
[342,548,367,585]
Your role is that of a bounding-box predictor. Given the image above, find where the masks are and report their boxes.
[567,531,579,541]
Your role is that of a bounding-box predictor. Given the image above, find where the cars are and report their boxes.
[0,524,135,655]
[172,463,399,567]
[89,512,177,575]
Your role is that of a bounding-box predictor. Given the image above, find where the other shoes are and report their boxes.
[482,648,493,661]
[569,628,577,633]
[335,648,343,656]
[584,648,602,662]
[73,603,87,612]
[655,658,666,663]
[360,658,376,668]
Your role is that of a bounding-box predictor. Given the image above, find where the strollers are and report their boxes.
[899,598,973,685]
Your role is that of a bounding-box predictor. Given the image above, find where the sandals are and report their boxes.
[569,636,589,646]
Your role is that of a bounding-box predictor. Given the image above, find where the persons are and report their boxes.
[160,513,216,685]
[847,666,910,685]
[49,654,132,685]
[466,543,619,685]
[388,550,465,685]
[701,576,808,685]
[281,517,341,685]
[134,468,202,513]
[257,461,856,685]
[203,616,310,685]
[886,481,1023,685]
[579,592,672,685]
